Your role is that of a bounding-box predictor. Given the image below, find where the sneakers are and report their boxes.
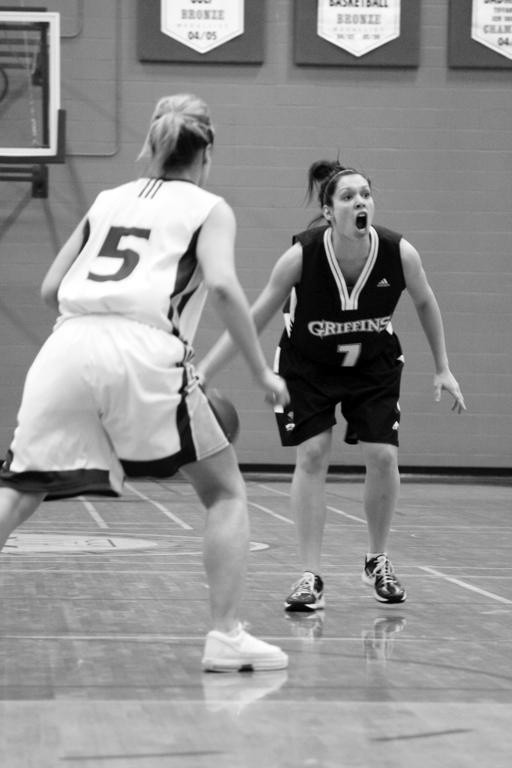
[361,553,407,604]
[201,623,289,672]
[284,571,325,612]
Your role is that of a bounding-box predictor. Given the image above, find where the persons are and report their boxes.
[0,94,289,673]
[194,159,467,611]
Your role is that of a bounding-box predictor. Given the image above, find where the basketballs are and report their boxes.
[204,387,238,444]
